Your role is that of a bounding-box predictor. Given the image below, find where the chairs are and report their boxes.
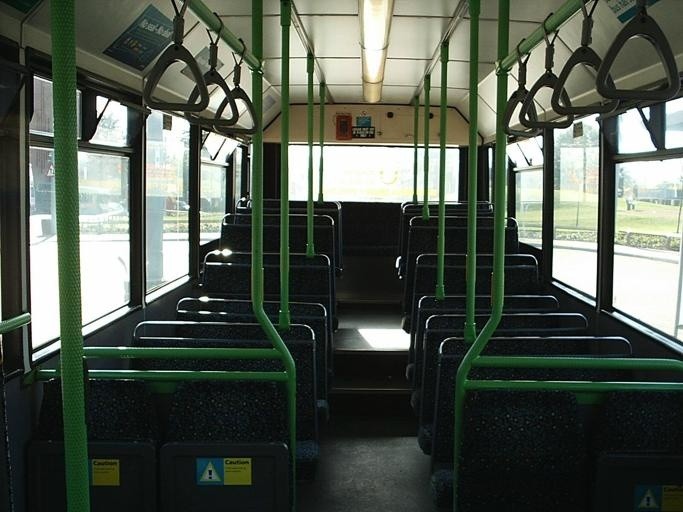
[22,185,683,511]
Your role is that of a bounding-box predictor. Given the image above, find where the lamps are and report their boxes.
[357,0,394,106]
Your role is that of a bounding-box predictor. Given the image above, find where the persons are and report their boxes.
[624,185,637,211]
